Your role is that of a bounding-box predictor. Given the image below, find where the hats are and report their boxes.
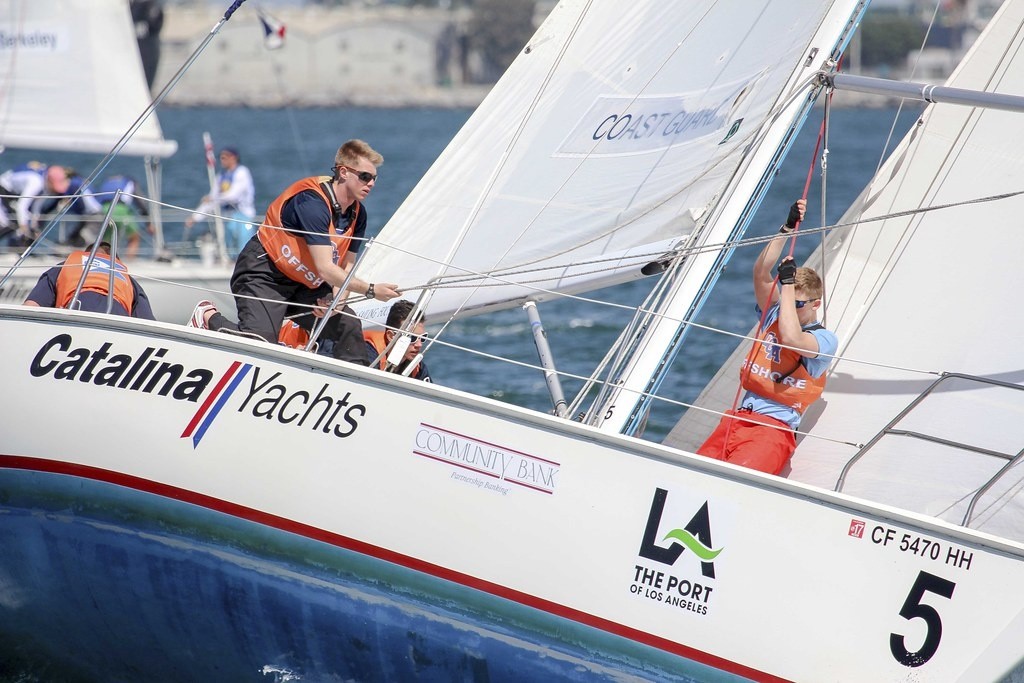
[49,167,70,193]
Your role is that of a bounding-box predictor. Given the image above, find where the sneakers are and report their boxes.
[186,299,217,329]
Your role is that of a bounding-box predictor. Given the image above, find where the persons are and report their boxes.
[277,299,432,384]
[0,161,154,259]
[185,137,401,365]
[21,241,157,320]
[184,147,256,261]
[694,199,838,476]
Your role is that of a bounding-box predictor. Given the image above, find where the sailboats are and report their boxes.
[2,0,312,330]
[0,0,1022,683]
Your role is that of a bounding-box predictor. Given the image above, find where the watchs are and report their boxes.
[365,283,375,299]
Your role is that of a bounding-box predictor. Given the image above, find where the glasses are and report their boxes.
[394,331,428,344]
[336,165,378,183]
[796,299,816,308]
